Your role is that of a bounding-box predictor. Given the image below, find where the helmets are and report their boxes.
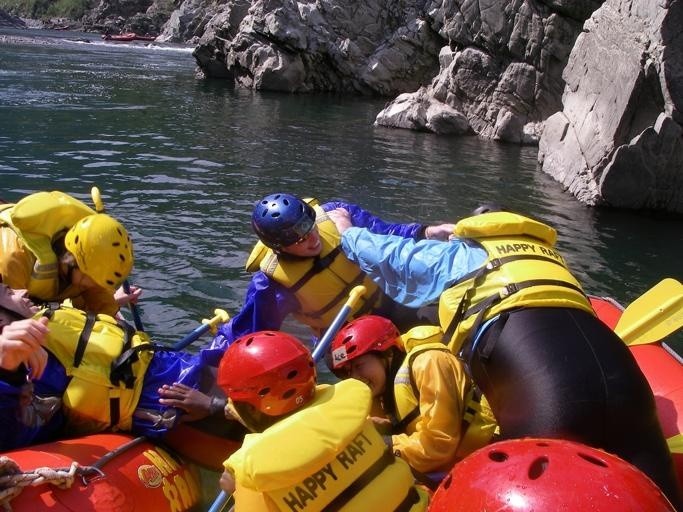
[217,330,318,416]
[428,438,676,512]
[251,192,316,250]
[331,315,405,369]
[64,213,135,293]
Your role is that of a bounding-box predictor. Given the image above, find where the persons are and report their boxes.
[0,191,505,512]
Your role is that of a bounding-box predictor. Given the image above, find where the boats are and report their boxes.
[101,33,155,41]
[2,294,683,511]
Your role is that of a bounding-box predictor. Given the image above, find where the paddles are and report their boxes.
[613,279,683,347]
